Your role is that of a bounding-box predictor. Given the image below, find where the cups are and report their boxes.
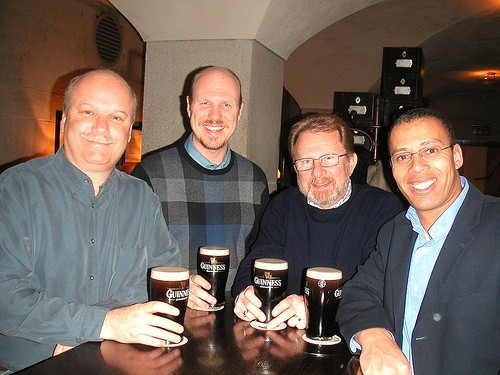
[252,258,288,326]
[198,246,230,307]
[304,267,343,341]
[149,267,189,343]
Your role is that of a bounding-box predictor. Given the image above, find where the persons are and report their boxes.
[230,114,404,328]
[129,65,270,309]
[335,108,500,375]
[0,70,185,375]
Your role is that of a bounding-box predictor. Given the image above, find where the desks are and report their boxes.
[456,139,500,195]
[9,297,362,375]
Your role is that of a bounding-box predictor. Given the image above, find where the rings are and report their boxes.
[293,314,301,322]
[242,309,249,317]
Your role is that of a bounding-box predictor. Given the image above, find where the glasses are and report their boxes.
[390,145,452,166]
[293,154,347,171]
